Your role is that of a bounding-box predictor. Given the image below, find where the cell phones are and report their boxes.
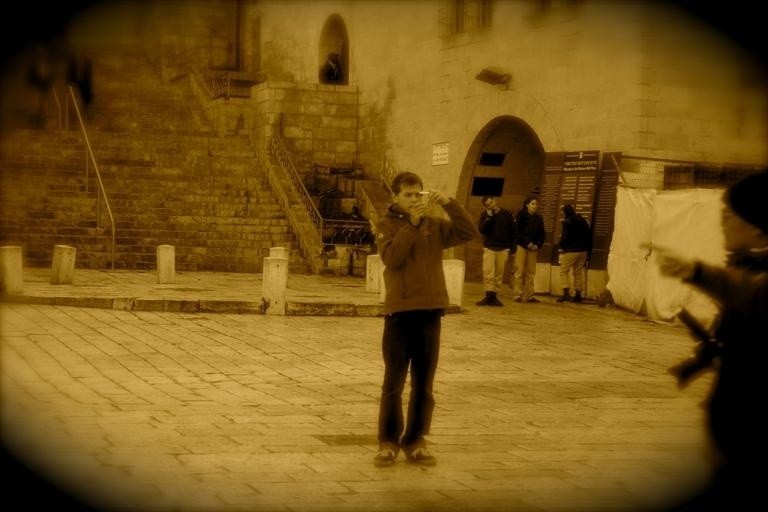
[419,192,430,218]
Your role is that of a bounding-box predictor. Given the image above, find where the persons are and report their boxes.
[641,163,767,512]
[474,195,514,306]
[65,47,95,121]
[25,47,56,129]
[317,52,344,85]
[554,204,592,304]
[371,169,479,464]
[511,196,545,302]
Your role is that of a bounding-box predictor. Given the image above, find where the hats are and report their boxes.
[722,168,767,233]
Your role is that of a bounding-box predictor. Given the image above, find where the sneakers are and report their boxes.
[374,450,396,465]
[406,447,437,465]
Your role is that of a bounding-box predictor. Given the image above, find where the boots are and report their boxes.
[477,288,583,306]
